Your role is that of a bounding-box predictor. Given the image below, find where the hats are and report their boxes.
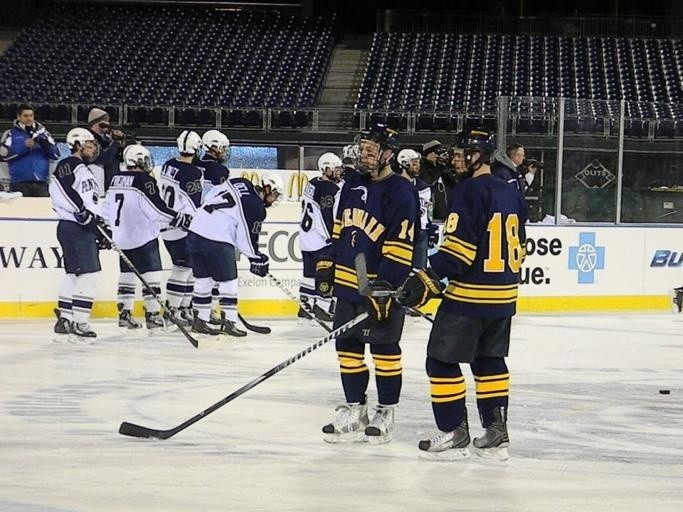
[87,108,112,129]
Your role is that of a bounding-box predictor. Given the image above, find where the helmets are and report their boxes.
[318,123,498,178]
[201,130,232,164]
[123,144,155,175]
[67,128,100,164]
[177,131,202,157]
[255,171,285,208]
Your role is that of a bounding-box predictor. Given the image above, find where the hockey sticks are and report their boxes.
[237,313,272,334]
[119,310,367,440]
[96,224,227,348]
[266,273,370,340]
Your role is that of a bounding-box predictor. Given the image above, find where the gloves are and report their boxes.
[96,224,113,250]
[35,133,50,150]
[249,256,269,277]
[397,269,447,309]
[171,213,193,232]
[314,257,335,298]
[366,282,395,321]
[76,210,100,228]
[426,223,439,250]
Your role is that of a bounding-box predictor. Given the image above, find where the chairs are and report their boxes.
[1,7,339,130]
[352,32,683,136]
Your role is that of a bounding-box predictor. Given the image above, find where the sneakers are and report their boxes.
[365,401,397,436]
[298,296,315,318]
[312,298,337,322]
[321,397,369,436]
[54,307,71,333]
[474,406,510,449]
[420,409,470,453]
[118,308,246,336]
[70,311,97,338]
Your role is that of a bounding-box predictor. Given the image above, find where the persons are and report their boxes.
[0,103,59,197]
[395,125,531,452]
[85,105,128,198]
[98,143,193,330]
[160,128,284,337]
[314,124,423,436]
[297,131,537,327]
[45,126,113,339]
